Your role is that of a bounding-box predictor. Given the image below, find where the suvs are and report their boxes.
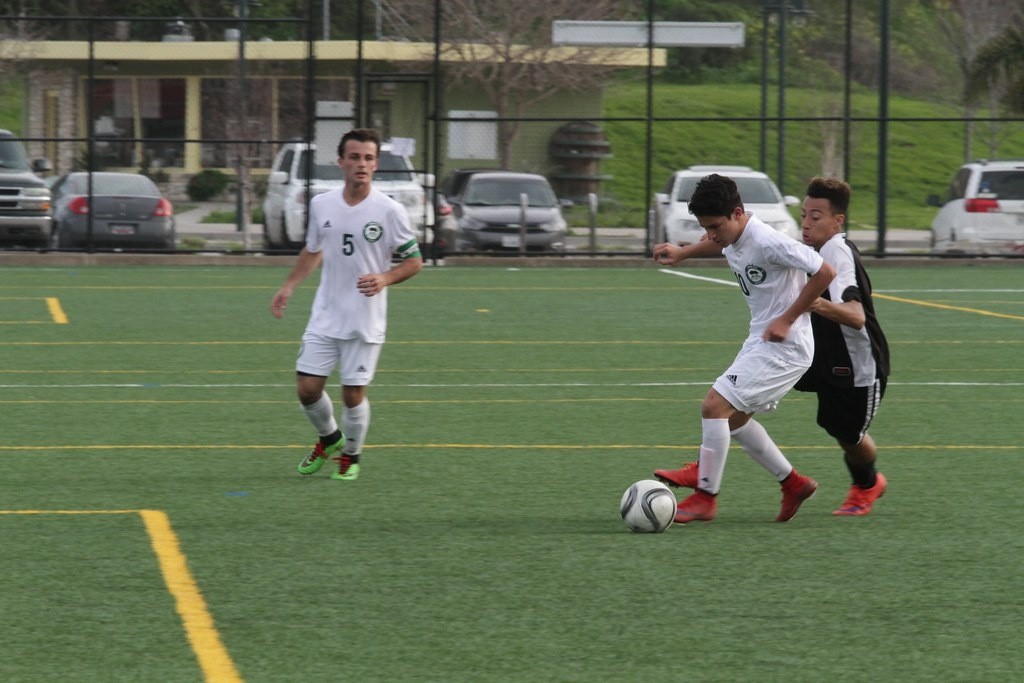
[262,137,438,256]
[0,128,54,249]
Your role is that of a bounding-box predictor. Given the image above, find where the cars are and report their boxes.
[434,192,458,254]
[924,158,1024,247]
[49,171,176,255]
[444,168,576,256]
[650,165,801,253]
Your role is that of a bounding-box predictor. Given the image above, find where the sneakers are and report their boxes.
[831,472,887,516]
[776,468,817,522]
[330,457,360,480]
[673,489,718,523]
[654,461,700,488]
[298,433,345,475]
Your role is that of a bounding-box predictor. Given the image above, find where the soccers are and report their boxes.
[620,479,678,533]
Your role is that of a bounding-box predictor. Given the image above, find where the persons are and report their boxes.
[270,128,423,481]
[653,173,836,524]
[651,174,892,515]
